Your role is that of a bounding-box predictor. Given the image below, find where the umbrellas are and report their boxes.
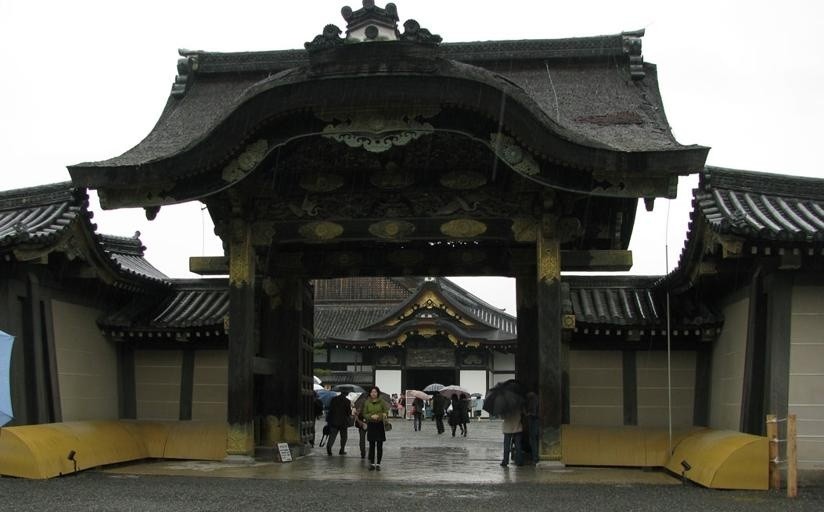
[482,380,529,416]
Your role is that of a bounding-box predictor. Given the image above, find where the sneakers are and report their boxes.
[500,461,508,466]
[368,464,382,471]
[339,451,347,454]
[326,448,332,455]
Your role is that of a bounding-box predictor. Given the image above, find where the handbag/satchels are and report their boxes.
[323,425,330,435]
[384,423,392,431]
[410,407,415,414]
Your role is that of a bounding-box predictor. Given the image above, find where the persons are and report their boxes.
[500,408,524,468]
[306,382,482,472]
[527,391,543,463]
